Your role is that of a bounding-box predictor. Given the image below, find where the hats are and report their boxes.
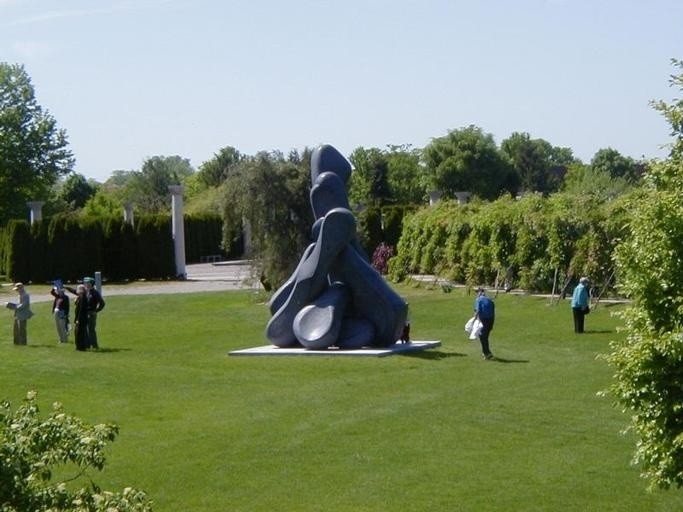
[13,282,26,291]
[474,287,484,291]
[83,278,95,285]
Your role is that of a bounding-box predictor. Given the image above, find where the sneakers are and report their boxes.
[480,352,494,361]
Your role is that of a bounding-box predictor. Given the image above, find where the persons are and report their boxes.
[11,281,33,345]
[570,276,590,334]
[71,284,90,351]
[474,287,495,359]
[60,277,105,349]
[50,284,72,345]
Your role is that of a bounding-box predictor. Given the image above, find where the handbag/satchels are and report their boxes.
[579,304,590,315]
